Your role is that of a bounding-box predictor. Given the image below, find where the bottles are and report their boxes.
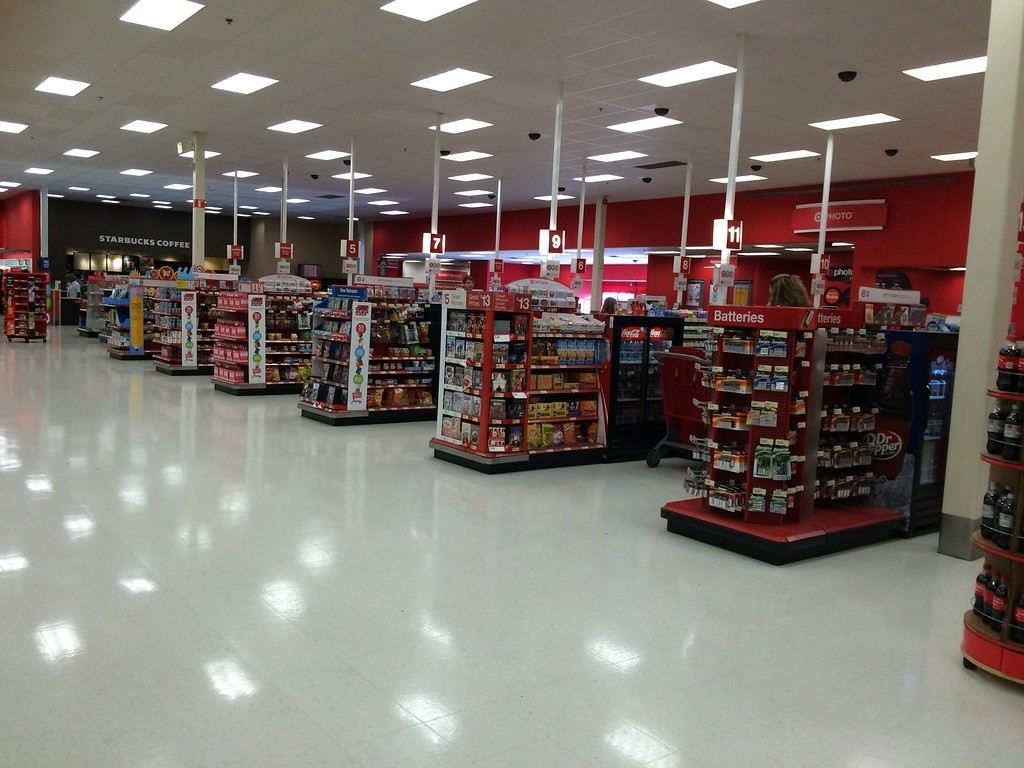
[615,341,669,422]
[981,481,1024,553]
[926,371,947,436]
[865,340,916,479]
[986,398,1024,461]
[974,564,1024,643]
[214,291,248,382]
[995,336,1024,394]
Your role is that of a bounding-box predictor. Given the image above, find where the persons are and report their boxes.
[601,297,618,314]
[766,273,814,311]
[428,304,442,404]
[461,274,476,291]
[69,274,81,297]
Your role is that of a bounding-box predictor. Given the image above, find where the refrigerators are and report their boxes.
[861,329,959,537]
[590,310,685,462]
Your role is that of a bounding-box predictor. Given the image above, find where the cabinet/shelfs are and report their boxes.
[150,285,197,376]
[265,291,327,395]
[105,281,143,361]
[142,284,156,360]
[198,288,220,376]
[963,366,1024,685]
[208,290,266,396]
[98,287,116,342]
[5,270,51,344]
[298,273,715,476]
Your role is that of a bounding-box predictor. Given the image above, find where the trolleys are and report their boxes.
[645,346,713,468]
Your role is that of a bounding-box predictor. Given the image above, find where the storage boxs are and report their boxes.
[538,403,541,418]
[448,309,457,331]
[457,310,466,332]
[580,400,597,416]
[569,402,576,416]
[577,315,605,333]
[567,339,576,364]
[446,336,456,358]
[473,397,481,417]
[489,398,506,420]
[514,315,527,333]
[494,320,510,334]
[211,290,249,382]
[594,340,600,364]
[567,402,570,414]
[530,375,536,390]
[492,371,508,392]
[445,365,454,384]
[564,382,579,389]
[552,403,558,418]
[529,403,538,419]
[456,339,464,359]
[455,366,463,385]
[542,312,575,332]
[477,314,486,334]
[471,424,479,444]
[553,373,564,389]
[492,343,509,363]
[462,394,472,416]
[475,341,485,362]
[443,416,452,437]
[563,373,566,382]
[564,386,570,389]
[537,374,552,390]
[444,391,452,410]
[463,367,472,389]
[576,401,580,414]
[540,403,552,419]
[466,314,477,333]
[557,339,567,365]
[559,313,591,332]
[531,355,558,365]
[453,392,462,413]
[508,425,522,445]
[452,418,460,440]
[558,403,568,417]
[577,340,584,365]
[461,422,470,442]
[472,369,482,390]
[510,343,527,363]
[507,399,525,418]
[465,340,474,363]
[566,372,569,382]
[577,372,597,388]
[488,426,506,447]
[509,370,525,392]
[586,340,593,364]
